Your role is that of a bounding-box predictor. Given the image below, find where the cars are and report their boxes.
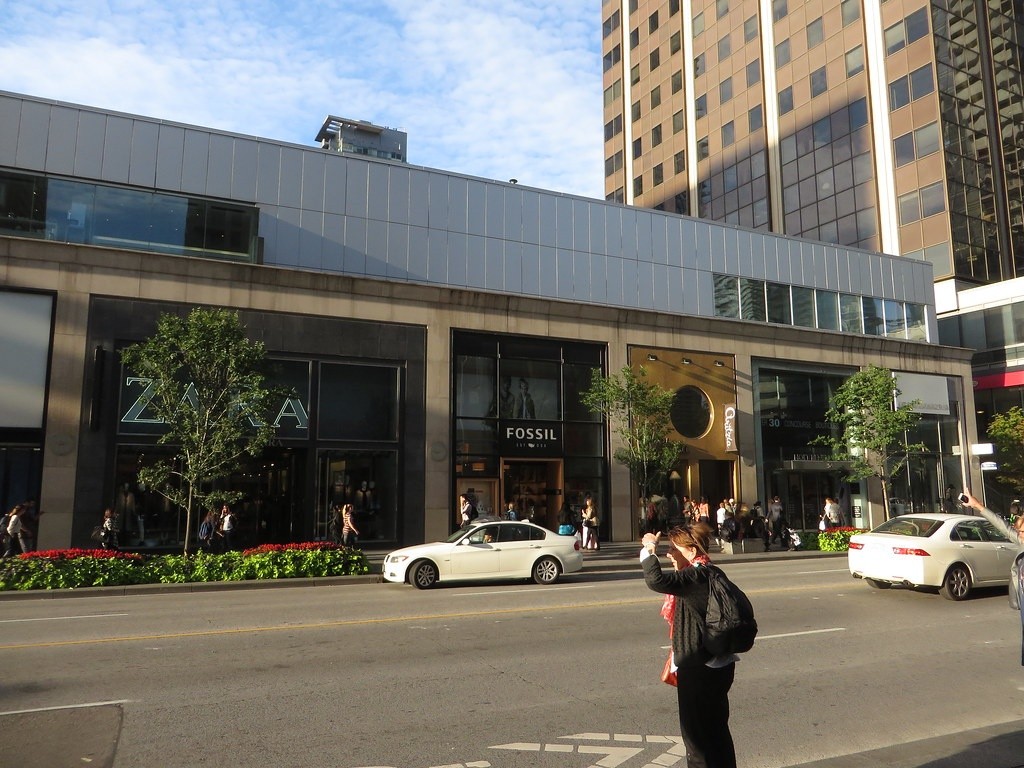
[848,513,1022,601]
[382,519,583,590]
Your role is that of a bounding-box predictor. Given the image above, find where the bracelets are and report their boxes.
[646,541,659,550]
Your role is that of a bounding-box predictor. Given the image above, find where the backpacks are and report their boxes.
[693,561,758,656]
[0,514,19,539]
[465,503,478,520]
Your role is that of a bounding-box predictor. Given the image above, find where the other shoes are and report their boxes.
[595,547,600,551]
[583,546,588,550]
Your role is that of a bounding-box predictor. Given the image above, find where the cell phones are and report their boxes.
[958,493,969,503]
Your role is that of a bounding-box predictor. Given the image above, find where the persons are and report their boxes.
[638,493,681,519]
[506,502,521,522]
[100,508,119,549]
[117,482,135,534]
[822,497,845,528]
[579,497,602,551]
[2,503,31,557]
[358,481,381,535]
[195,511,220,552]
[557,502,580,536]
[639,522,741,768]
[341,504,360,550]
[218,502,239,554]
[680,496,710,524]
[484,529,495,542]
[963,486,1024,667]
[161,483,174,539]
[459,494,472,528]
[717,495,790,552]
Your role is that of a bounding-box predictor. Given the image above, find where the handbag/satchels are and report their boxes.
[583,520,591,527]
[660,656,678,687]
[92,519,114,544]
